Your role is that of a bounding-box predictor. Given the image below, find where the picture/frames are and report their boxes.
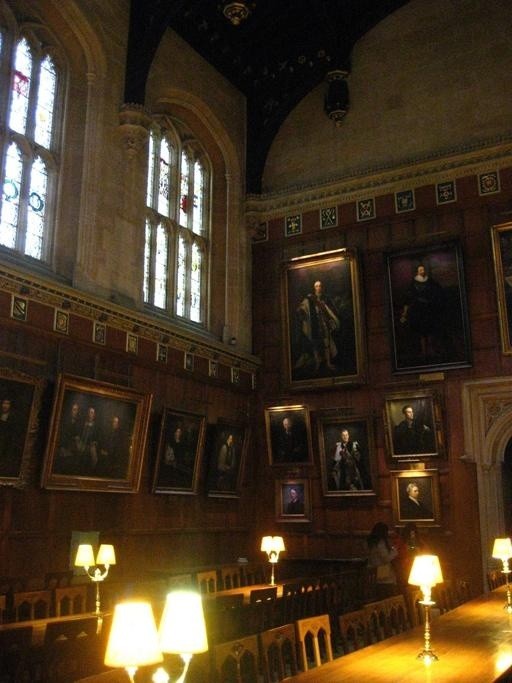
[488,219,511,356]
[37,364,154,494]
[261,388,446,530]
[382,227,475,377]
[205,419,255,500]
[278,244,369,394]
[0,366,53,492]
[148,405,208,497]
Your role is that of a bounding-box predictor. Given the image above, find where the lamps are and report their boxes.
[74,541,117,616]
[258,535,287,586]
[491,536,512,573]
[408,550,447,663]
[323,56,352,130]
[103,590,207,680]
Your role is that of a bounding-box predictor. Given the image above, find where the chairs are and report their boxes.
[0,550,512,681]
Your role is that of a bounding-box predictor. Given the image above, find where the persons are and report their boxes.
[392,403,431,455]
[100,414,128,478]
[215,431,242,491]
[286,488,304,512]
[391,522,428,588]
[399,481,431,518]
[74,404,100,475]
[55,399,81,474]
[274,417,305,460]
[158,425,185,485]
[330,428,367,488]
[0,395,19,472]
[399,262,451,363]
[364,522,402,598]
[295,278,346,377]
